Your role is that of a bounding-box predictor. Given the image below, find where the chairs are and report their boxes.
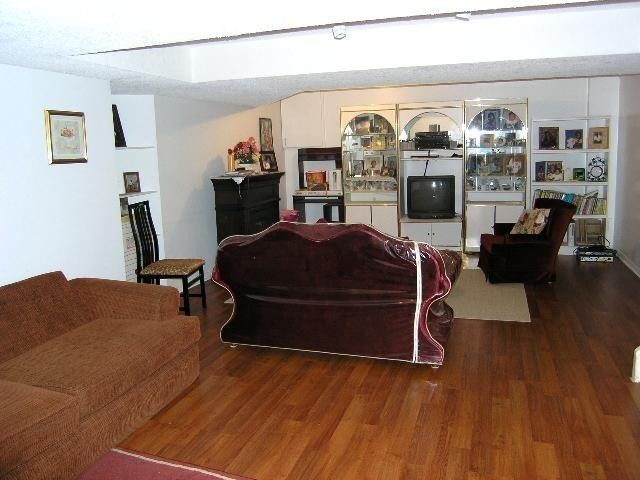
[125,200,208,319]
[476,196,577,289]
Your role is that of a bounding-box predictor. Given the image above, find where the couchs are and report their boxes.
[0,266,205,480]
[209,217,464,374]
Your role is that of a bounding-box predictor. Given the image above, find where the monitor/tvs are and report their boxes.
[407,176,455,219]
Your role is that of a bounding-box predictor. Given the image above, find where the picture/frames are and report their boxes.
[44,109,89,165]
[348,115,396,177]
[257,150,280,173]
[588,125,611,150]
[111,102,127,148]
[123,171,142,194]
[467,108,527,178]
[258,116,275,152]
[538,125,560,152]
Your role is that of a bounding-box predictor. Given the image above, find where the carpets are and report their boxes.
[222,268,533,324]
[69,446,259,479]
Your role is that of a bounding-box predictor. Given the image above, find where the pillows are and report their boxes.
[509,206,550,237]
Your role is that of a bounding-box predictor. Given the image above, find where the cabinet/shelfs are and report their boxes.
[463,97,529,258]
[340,104,399,239]
[530,113,615,255]
[280,91,324,149]
[396,100,465,258]
[209,168,286,248]
[115,143,162,239]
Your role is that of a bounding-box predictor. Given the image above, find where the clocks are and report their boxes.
[585,156,608,183]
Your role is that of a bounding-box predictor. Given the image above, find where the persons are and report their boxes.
[542,132,553,146]
[504,113,520,129]
[550,169,562,181]
[485,114,495,129]
[575,132,583,143]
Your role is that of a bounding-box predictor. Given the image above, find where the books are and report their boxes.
[532,189,606,214]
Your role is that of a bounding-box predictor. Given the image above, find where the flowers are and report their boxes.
[231,133,263,164]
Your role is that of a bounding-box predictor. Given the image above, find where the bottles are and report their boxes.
[467,149,524,192]
[227,154,236,172]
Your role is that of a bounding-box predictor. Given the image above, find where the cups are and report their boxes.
[346,167,398,192]
[226,170,255,177]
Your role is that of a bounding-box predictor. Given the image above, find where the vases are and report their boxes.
[236,162,260,175]
